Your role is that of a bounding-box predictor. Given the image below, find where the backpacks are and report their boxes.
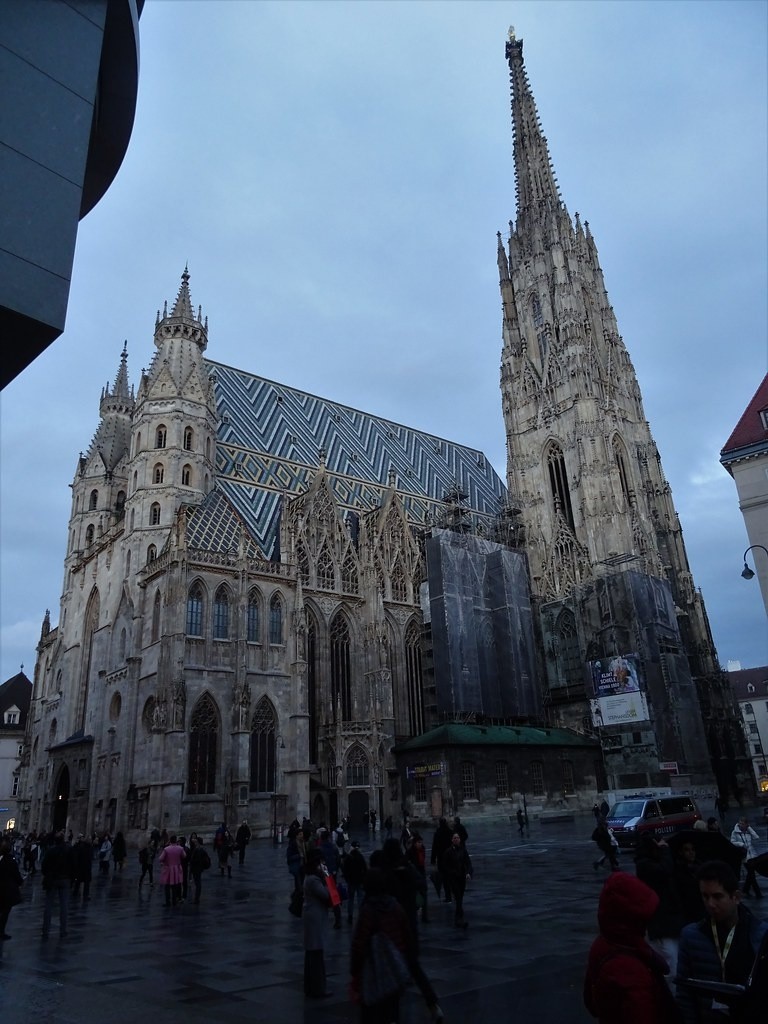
[139,849,147,863]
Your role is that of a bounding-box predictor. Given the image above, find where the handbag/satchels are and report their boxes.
[325,875,341,906]
[200,848,211,870]
[287,895,304,918]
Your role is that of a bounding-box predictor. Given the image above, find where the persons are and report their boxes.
[674,859,768,1024]
[583,869,687,1024]
[0,767,768,1024]
[112,831,126,870]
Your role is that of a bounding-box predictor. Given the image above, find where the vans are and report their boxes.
[606,795,702,847]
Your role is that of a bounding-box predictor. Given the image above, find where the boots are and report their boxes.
[189,894,201,904]
[227,864,233,878]
[221,867,224,878]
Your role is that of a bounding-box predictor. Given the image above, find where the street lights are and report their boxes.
[738,700,767,775]
[274,735,285,844]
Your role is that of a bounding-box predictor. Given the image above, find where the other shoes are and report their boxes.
[742,889,753,897]
[757,894,765,900]
[40,930,48,938]
[431,1004,444,1024]
[83,894,92,901]
[443,898,452,904]
[305,988,333,999]
[334,921,342,930]
[347,917,353,924]
[593,861,598,871]
[162,903,171,907]
[0,934,12,940]
[422,918,431,923]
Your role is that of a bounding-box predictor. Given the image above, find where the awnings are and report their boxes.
[394,723,598,751]
